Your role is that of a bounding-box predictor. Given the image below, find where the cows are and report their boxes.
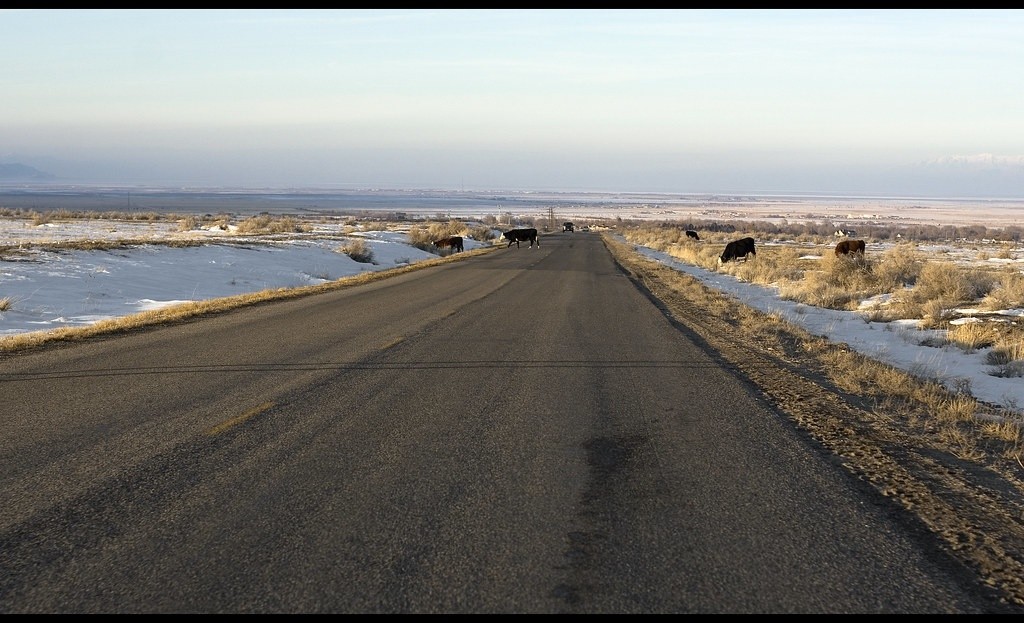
[685,230,700,240]
[435,236,464,254]
[835,239,866,260]
[499,228,540,250]
[719,237,757,265]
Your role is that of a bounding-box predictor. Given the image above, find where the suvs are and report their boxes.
[563,222,574,234]
